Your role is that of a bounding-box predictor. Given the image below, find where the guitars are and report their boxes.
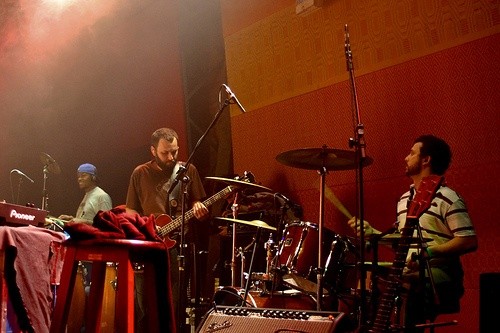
[155,171,259,251]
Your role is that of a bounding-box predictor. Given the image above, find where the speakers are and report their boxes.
[194,306,353,333]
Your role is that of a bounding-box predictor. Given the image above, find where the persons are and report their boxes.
[124,128,210,333]
[346,135,478,326]
[58,163,112,226]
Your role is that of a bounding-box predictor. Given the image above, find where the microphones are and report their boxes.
[344,25,353,71]
[14,169,34,183]
[279,192,300,210]
[223,83,246,112]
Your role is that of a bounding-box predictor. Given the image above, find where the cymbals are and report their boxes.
[274,147,375,172]
[204,175,274,193]
[214,215,278,233]
[362,234,436,245]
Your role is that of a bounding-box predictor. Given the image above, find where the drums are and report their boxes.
[350,261,403,297]
[96,261,117,332]
[268,221,356,299]
[211,285,323,313]
[54,262,87,332]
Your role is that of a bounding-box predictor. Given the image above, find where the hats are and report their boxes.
[78,163,96,175]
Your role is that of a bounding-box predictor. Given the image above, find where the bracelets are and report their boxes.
[426,248,432,257]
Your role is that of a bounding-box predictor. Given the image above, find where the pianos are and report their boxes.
[0,200,49,228]
